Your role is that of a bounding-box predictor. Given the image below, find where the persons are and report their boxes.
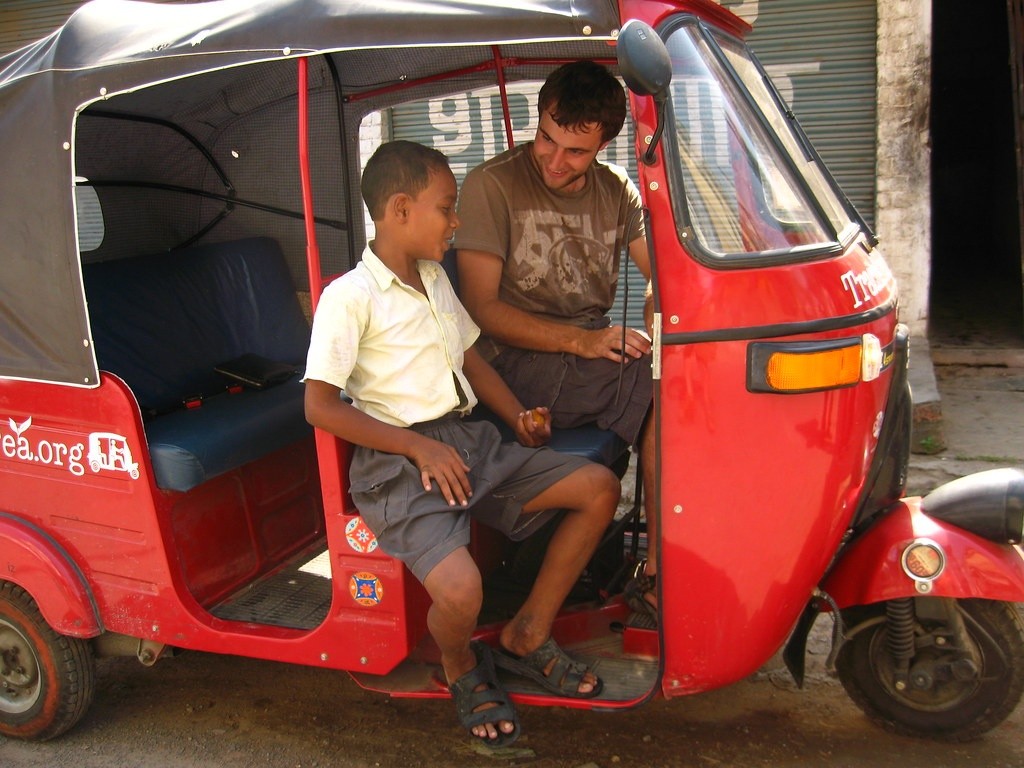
[445,55,658,607]
[300,140,625,757]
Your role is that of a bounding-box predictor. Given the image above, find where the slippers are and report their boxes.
[440,651,520,747]
[492,634,604,699]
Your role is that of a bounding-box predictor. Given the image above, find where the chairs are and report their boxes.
[418,246,630,526]
[81,229,321,496]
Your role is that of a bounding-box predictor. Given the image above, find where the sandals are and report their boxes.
[623,558,657,628]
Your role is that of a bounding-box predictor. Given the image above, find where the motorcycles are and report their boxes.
[0,0,1024,740]
